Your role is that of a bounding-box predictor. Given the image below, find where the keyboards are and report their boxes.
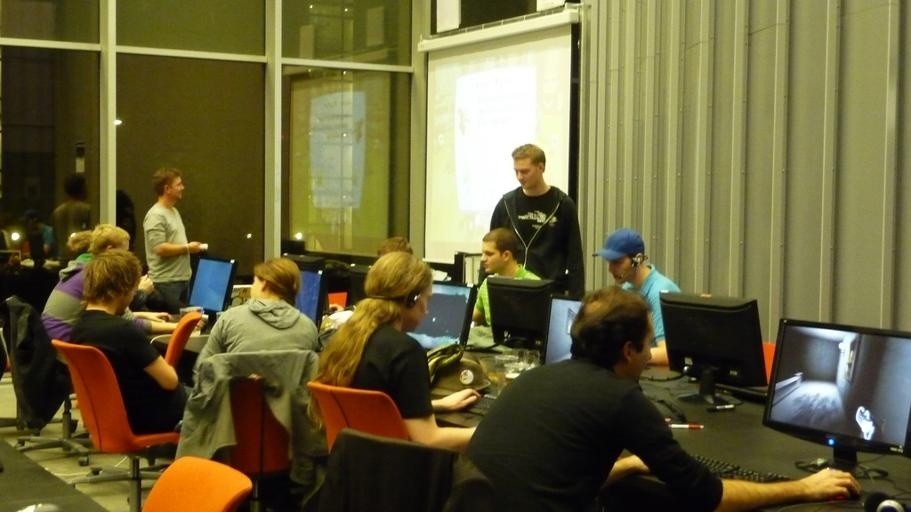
[466,394,495,414]
[677,452,789,484]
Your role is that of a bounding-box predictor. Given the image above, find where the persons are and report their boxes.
[40,223,208,371]
[190,257,321,396]
[487,143,587,301]
[590,227,685,366]
[69,247,194,434]
[141,167,206,316]
[37,222,93,306]
[10,209,56,301]
[471,225,544,326]
[304,248,482,453]
[49,172,96,264]
[440,282,865,512]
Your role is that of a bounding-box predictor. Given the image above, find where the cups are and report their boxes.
[495,355,518,397]
[503,347,525,379]
[524,350,540,369]
[180,308,203,337]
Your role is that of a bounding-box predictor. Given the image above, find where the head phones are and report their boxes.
[364,288,421,307]
[863,493,910,512]
[630,255,647,267]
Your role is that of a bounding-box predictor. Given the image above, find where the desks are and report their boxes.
[417,347,911,512]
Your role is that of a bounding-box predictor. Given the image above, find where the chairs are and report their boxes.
[306,378,414,458]
[139,452,254,512]
[48,335,183,511]
[2,296,96,467]
[209,350,322,480]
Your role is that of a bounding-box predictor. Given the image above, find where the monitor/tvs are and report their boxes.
[187,256,236,313]
[488,278,554,345]
[762,318,910,479]
[298,264,328,331]
[544,296,582,364]
[660,294,767,405]
[404,281,478,348]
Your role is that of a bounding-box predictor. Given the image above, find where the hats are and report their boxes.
[429,359,491,397]
[592,228,645,261]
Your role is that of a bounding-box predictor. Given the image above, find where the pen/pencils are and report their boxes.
[707,404,737,412]
[481,393,498,400]
[670,424,704,430]
